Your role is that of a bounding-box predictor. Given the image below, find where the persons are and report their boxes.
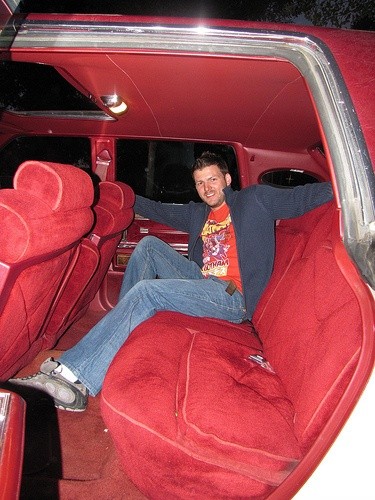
[7,151,336,412]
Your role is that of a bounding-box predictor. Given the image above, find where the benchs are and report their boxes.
[98,192,375,500]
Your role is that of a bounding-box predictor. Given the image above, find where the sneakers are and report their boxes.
[7,357,88,412]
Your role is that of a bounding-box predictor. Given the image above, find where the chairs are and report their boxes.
[0,161,97,385]
[34,182,134,350]
